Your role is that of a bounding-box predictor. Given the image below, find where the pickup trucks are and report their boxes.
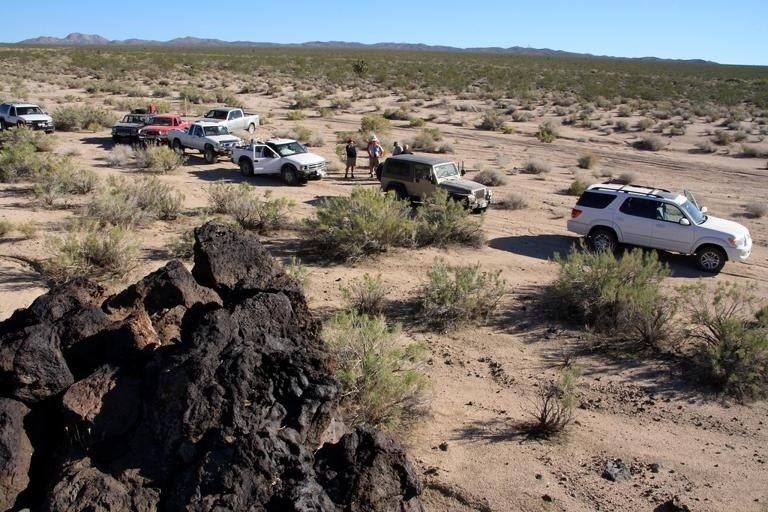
[111,110,192,148]
[165,121,244,163]
[223,137,325,186]
[195,107,259,135]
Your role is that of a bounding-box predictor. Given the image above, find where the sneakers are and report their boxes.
[343,174,355,178]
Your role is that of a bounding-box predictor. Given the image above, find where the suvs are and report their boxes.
[376,154,490,215]
[568,181,753,274]
[0,101,55,134]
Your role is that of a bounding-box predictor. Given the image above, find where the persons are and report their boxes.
[367,134,380,174]
[149,97,157,113]
[368,137,384,179]
[400,143,414,155]
[392,141,402,155]
[343,138,358,179]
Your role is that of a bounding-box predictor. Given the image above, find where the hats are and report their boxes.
[347,138,355,143]
[371,137,377,142]
